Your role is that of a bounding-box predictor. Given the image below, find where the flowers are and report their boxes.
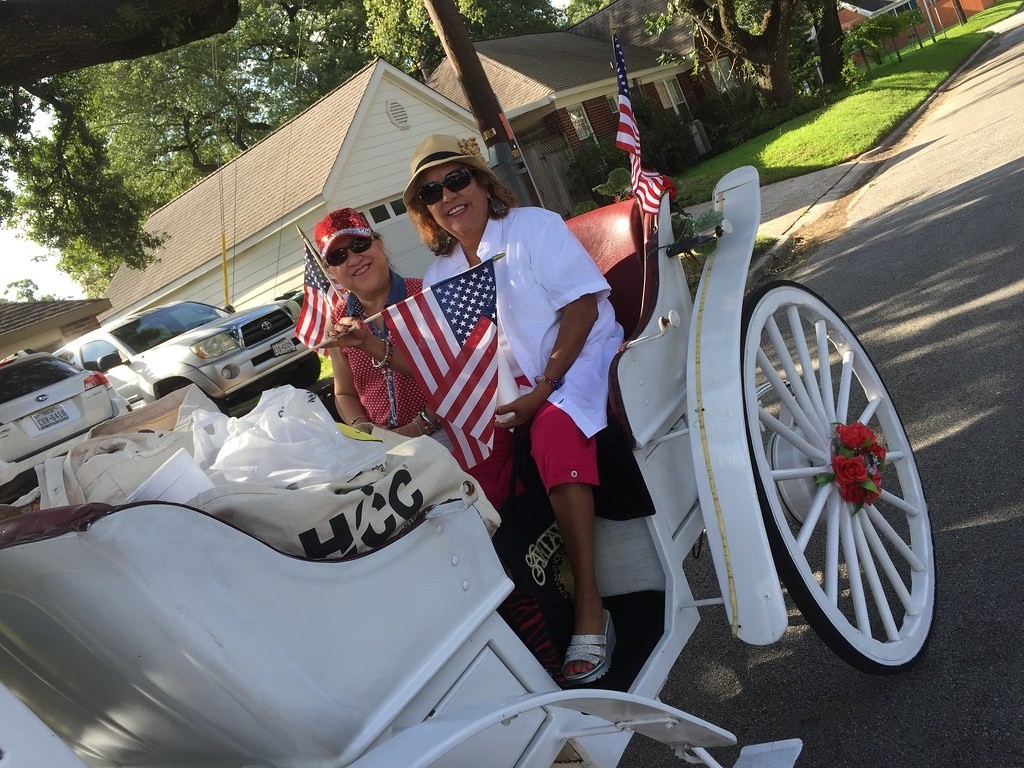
[812,421,895,518]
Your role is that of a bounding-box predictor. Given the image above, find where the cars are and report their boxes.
[0,348,133,513]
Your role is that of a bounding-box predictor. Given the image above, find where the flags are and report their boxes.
[379,257,495,468]
[612,34,675,213]
[294,243,352,353]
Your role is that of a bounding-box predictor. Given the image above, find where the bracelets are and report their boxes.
[410,409,442,437]
[372,339,389,368]
[535,375,564,389]
[348,414,375,429]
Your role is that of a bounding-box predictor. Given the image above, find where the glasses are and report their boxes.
[326,234,377,266]
[417,167,472,206]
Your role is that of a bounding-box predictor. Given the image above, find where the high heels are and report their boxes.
[562,609,617,684]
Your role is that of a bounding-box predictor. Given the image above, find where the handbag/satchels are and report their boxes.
[491,426,576,658]
[34,432,193,511]
[193,385,386,489]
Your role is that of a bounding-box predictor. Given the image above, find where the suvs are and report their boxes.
[52,300,321,415]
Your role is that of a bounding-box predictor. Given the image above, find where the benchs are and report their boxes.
[2,498,514,768]
[560,195,690,451]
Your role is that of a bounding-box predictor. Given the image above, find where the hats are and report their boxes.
[402,133,498,208]
[313,207,377,260]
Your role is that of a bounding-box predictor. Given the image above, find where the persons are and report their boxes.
[315,207,463,456]
[401,134,624,684]
